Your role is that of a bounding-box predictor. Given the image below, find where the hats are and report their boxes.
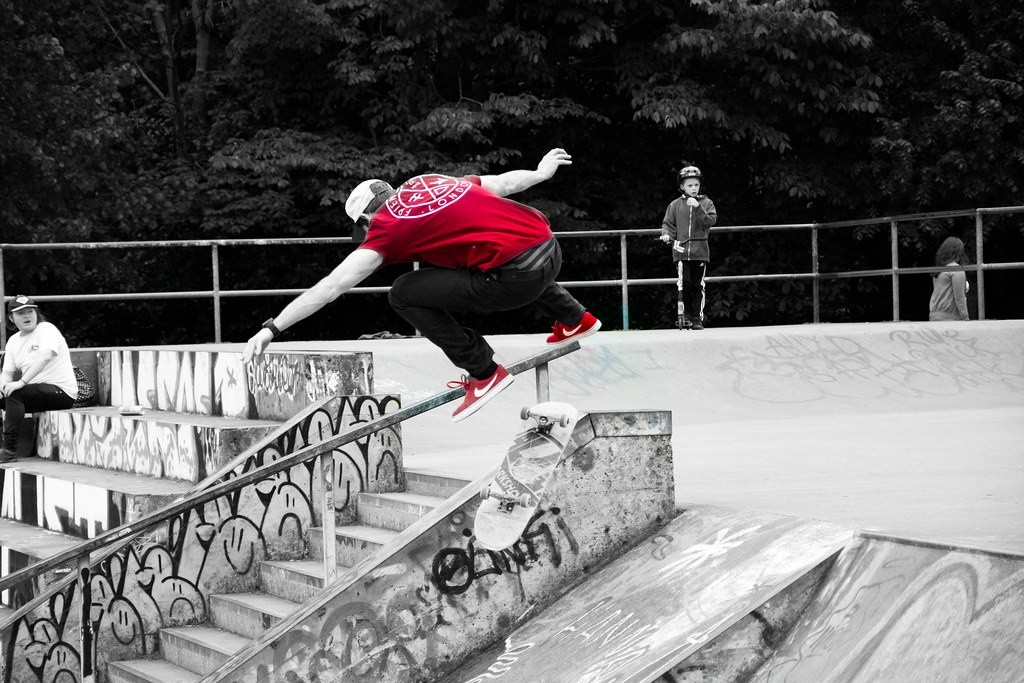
[345,178,393,242]
[8,292,39,314]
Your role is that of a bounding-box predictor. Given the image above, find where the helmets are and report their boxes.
[675,164,704,183]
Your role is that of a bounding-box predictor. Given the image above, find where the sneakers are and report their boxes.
[446,363,514,423]
[544,309,602,351]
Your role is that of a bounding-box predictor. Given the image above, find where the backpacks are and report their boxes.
[72,365,96,407]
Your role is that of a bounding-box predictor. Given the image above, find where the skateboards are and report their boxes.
[472,401,579,552]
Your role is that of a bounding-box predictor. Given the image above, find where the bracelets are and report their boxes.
[21,379,26,385]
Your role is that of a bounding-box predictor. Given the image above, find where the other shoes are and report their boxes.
[0,447,19,464]
[690,314,704,330]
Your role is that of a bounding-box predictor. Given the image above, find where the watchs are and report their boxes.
[260,317,282,337]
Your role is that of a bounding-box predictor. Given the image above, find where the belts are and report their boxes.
[497,242,561,285]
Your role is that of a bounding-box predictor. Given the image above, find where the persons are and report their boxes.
[659,166,717,330]
[930,238,970,320]
[0,295,78,465]
[241,147,602,425]
